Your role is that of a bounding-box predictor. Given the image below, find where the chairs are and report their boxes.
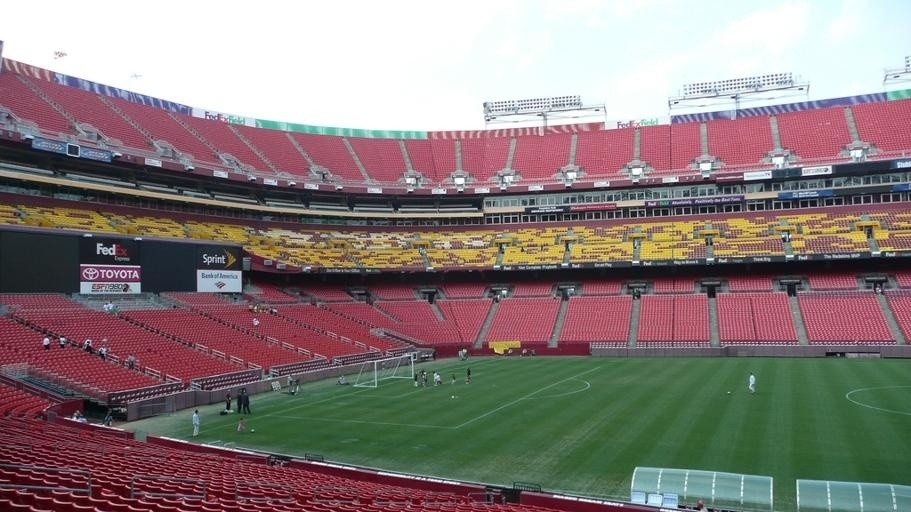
[0,279,427,413]
[0,414,569,512]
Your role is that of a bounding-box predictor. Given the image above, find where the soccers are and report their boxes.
[452,396,455,399]
[728,391,730,394]
[251,429,256,433]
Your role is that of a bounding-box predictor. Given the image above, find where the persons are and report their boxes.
[461,347,468,361]
[67,410,87,424]
[103,413,113,427]
[99,344,107,361]
[248,303,277,316]
[128,354,135,371]
[507,348,537,361]
[59,334,67,349]
[239,417,245,433]
[103,300,121,318]
[192,410,201,439]
[696,500,709,512]
[287,373,304,393]
[748,372,756,394]
[224,388,251,414]
[42,336,51,350]
[412,367,472,388]
